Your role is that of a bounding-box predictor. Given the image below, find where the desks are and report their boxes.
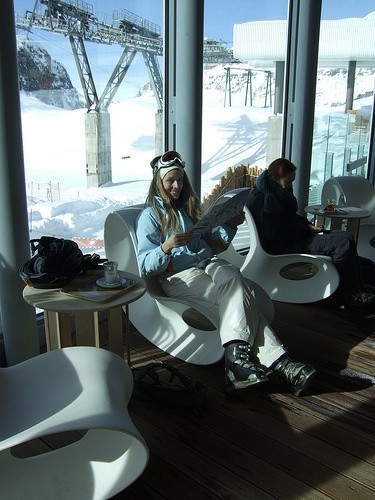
[304,204,370,246]
[19,269,147,355]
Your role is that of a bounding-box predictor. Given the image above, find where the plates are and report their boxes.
[96,277,126,288]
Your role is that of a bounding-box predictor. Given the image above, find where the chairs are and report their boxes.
[0,175,375,500]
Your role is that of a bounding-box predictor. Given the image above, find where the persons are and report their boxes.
[248,159,375,316]
[135,151,316,397]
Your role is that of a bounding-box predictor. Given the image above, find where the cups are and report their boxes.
[327,202,335,212]
[102,261,118,286]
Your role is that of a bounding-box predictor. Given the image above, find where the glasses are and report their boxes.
[152,151,186,168]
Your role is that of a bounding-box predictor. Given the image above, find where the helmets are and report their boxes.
[18,235,83,289]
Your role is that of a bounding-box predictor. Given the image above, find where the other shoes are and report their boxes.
[277,356,318,393]
[336,293,360,313]
[224,344,279,394]
